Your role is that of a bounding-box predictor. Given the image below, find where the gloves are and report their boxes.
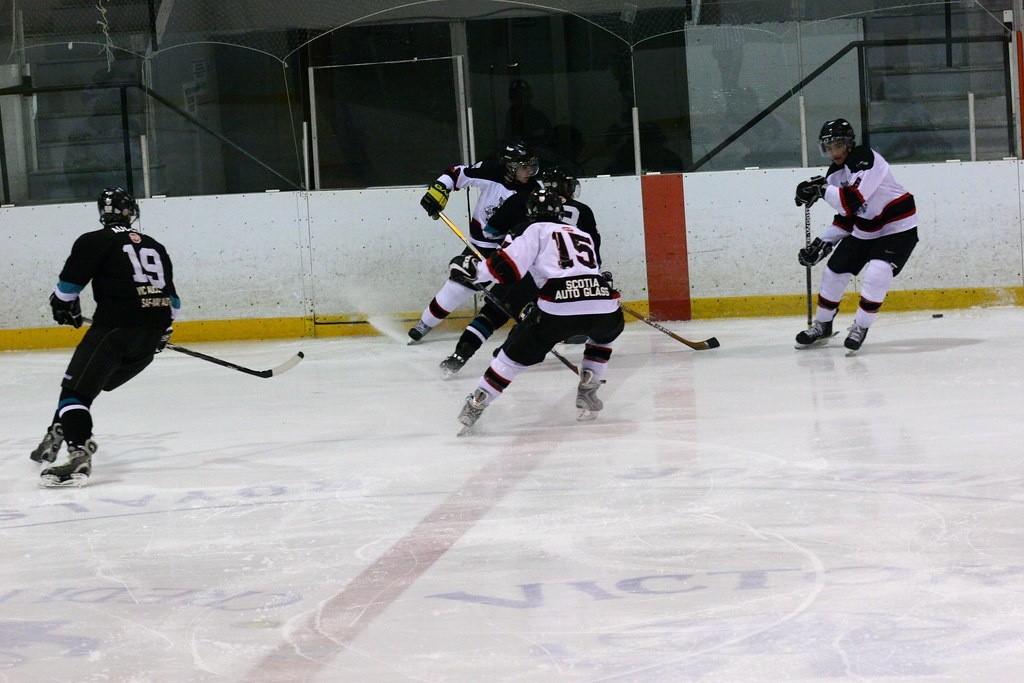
[602,271,613,288]
[795,175,827,207]
[155,328,173,354]
[49,292,84,329]
[449,256,479,285]
[421,181,452,221]
[798,238,834,267]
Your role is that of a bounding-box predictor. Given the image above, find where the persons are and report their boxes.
[405,141,539,346]
[438,166,602,382]
[792,116,919,357]
[28,190,180,486]
[446,187,624,439]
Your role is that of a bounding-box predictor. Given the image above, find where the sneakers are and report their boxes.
[458,387,491,436]
[31,409,63,463]
[407,319,432,345]
[795,308,838,350]
[844,319,869,357]
[440,343,470,378]
[576,369,605,421]
[39,439,97,487]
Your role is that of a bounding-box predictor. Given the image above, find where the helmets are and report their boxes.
[527,189,564,224]
[819,119,855,153]
[97,187,135,223]
[540,167,576,197]
[499,140,538,175]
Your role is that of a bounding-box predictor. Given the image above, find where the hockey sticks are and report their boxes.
[81,314,306,379]
[439,212,589,344]
[474,281,579,375]
[805,199,841,337]
[615,302,721,351]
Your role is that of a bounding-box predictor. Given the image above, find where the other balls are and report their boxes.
[932,313,944,319]
